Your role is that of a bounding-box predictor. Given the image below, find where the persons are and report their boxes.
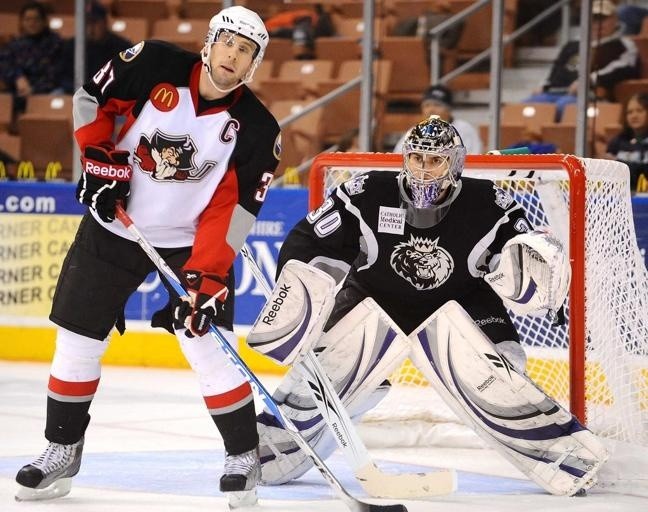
[607,95,648,192]
[246,116,614,495]
[393,85,482,155]
[263,0,462,61]
[2,1,135,96]
[539,0,639,100]
[15,6,281,510]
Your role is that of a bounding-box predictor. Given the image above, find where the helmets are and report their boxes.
[199,5,270,82]
[395,114,468,229]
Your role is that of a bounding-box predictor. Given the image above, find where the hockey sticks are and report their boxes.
[237,239,455,501]
[112,204,457,512]
[111,202,409,512]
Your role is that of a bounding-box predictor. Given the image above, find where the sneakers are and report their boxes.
[218,448,261,491]
[16,439,91,491]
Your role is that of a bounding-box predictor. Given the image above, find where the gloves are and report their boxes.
[170,269,231,338]
[74,143,133,222]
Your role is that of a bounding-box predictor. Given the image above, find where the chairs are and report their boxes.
[0,0,219,47]
[232,2,646,184]
[2,93,75,180]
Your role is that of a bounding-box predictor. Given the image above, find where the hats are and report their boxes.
[591,0,616,18]
[421,84,451,107]
